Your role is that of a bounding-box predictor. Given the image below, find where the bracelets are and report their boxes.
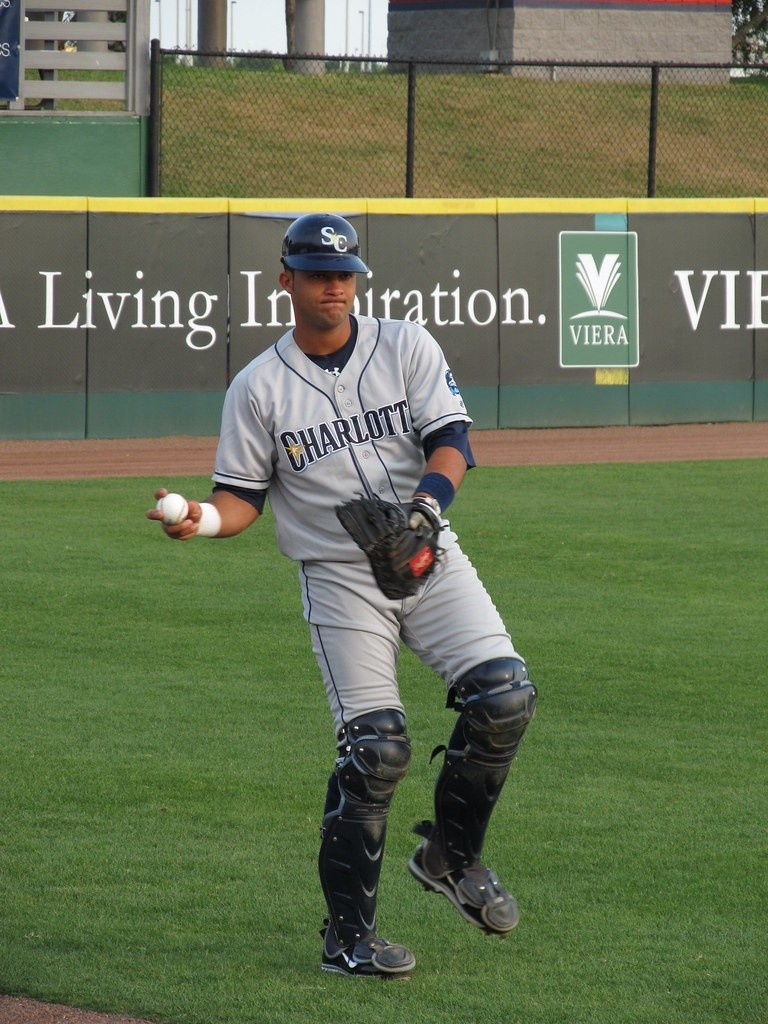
[196,503,221,538]
[412,473,456,515]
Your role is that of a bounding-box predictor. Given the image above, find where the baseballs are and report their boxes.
[157,495,188,524]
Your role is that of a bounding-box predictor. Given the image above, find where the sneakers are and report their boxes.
[408,839,519,935]
[321,924,416,980]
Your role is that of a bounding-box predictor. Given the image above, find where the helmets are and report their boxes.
[280,213,369,273]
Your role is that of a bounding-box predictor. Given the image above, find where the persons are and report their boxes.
[147,213,538,979]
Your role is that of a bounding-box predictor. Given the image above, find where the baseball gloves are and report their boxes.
[333,491,442,599]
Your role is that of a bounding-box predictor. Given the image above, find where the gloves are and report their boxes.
[409,496,442,538]
